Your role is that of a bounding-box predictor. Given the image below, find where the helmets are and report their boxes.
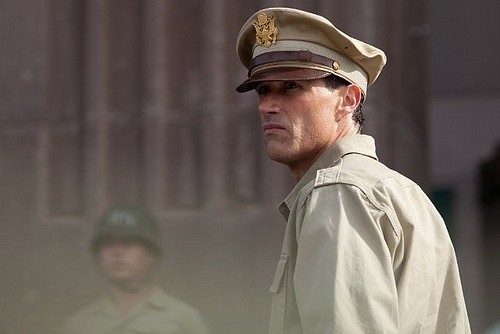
[91,204,166,263]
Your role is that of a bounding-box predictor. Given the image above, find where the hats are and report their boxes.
[235,7,387,101]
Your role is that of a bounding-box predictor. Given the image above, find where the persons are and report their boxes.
[54,199,210,334]
[236,6,473,334]
